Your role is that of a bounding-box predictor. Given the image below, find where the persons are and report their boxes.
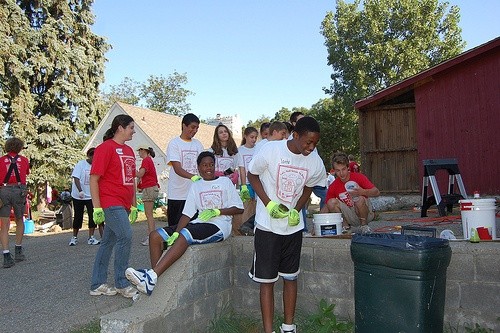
[135,143,160,245]
[166,113,204,227]
[0,138,30,267]
[247,117,324,333]
[311,153,381,236]
[50,147,105,246]
[125,151,245,296]
[205,111,359,235]
[89,114,139,297]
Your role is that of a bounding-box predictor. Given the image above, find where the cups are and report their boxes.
[345,181,360,202]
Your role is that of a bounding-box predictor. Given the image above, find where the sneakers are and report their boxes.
[125,268,157,296]
[69,236,78,246]
[88,236,100,245]
[90,283,118,296]
[114,286,139,297]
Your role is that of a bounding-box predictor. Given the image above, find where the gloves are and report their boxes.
[288,209,300,226]
[191,175,201,182]
[128,207,138,224]
[92,208,105,224]
[240,185,250,202]
[198,208,220,221]
[79,191,84,199]
[266,201,288,219]
[166,232,179,246]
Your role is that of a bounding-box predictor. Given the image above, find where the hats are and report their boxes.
[135,144,150,151]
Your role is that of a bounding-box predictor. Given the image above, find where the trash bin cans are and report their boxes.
[351,226,452,333]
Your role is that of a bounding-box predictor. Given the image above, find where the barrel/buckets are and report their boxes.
[459,198,497,241]
[312,212,343,236]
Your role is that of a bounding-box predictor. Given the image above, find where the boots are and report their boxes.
[14,246,25,262]
[3,252,14,268]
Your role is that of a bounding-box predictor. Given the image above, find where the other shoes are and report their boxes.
[279,323,296,333]
[342,226,351,233]
[361,224,370,233]
[140,236,149,246]
